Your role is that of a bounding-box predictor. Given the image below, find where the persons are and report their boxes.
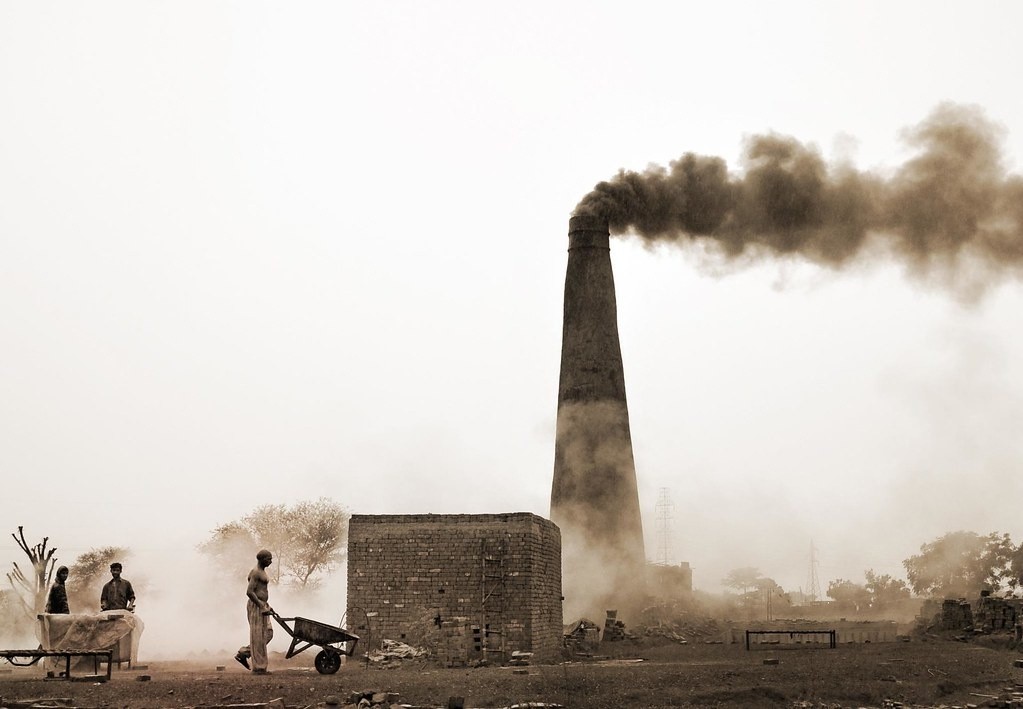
[45,566,69,678]
[235,550,273,675]
[100,562,136,611]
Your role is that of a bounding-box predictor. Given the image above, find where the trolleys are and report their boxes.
[264,609,360,675]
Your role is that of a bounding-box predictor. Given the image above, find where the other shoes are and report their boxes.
[251,669,272,675]
[235,653,251,670]
